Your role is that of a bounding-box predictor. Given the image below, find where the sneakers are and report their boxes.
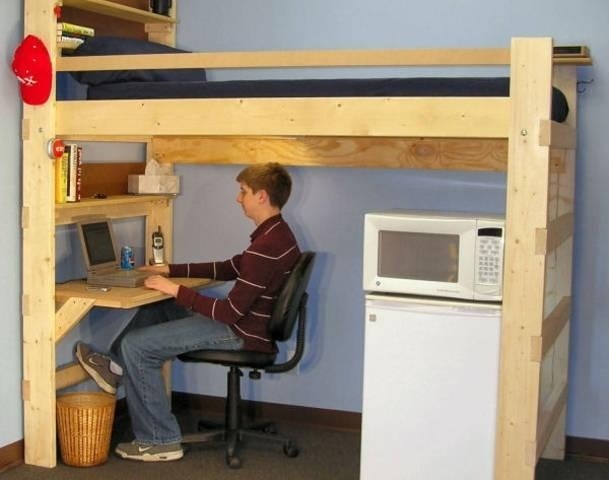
[74,340,123,394]
[115,440,185,462]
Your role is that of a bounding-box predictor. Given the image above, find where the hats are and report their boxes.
[12,35,52,105]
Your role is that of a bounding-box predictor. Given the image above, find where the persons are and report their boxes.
[73,162,302,463]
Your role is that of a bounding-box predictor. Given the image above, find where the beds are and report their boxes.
[23,2,590,476]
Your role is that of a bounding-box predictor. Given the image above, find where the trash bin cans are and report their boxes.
[57,391,116,467]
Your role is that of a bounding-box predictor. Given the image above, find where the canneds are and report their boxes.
[121,246,135,270]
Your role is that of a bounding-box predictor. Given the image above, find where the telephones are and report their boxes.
[149,225,168,268]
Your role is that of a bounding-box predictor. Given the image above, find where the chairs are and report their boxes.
[170,250,321,469]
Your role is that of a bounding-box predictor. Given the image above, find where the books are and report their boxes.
[56,22,95,42]
[55,143,82,205]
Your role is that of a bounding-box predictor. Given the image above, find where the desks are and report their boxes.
[23,265,228,466]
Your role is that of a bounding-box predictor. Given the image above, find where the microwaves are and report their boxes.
[361,208,505,305]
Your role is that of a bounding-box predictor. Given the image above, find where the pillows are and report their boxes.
[71,38,209,83]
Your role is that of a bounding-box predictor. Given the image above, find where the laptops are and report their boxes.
[76,217,170,288]
